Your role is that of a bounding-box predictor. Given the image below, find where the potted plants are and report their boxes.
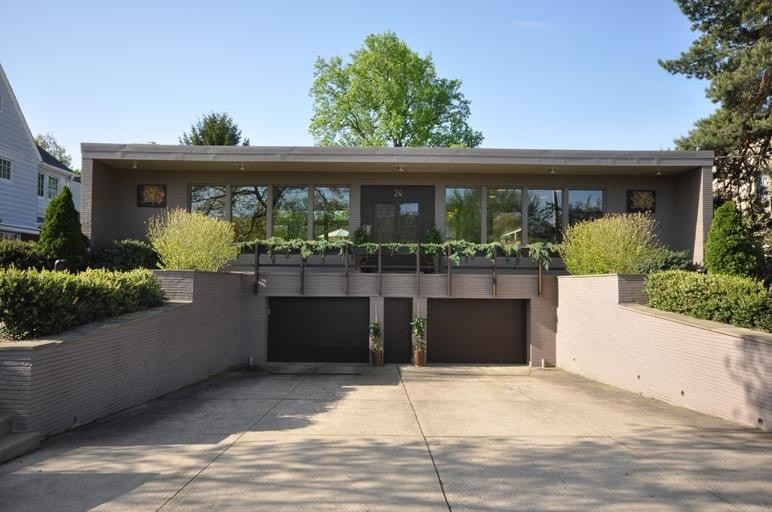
[412,317,425,367]
[370,323,384,366]
